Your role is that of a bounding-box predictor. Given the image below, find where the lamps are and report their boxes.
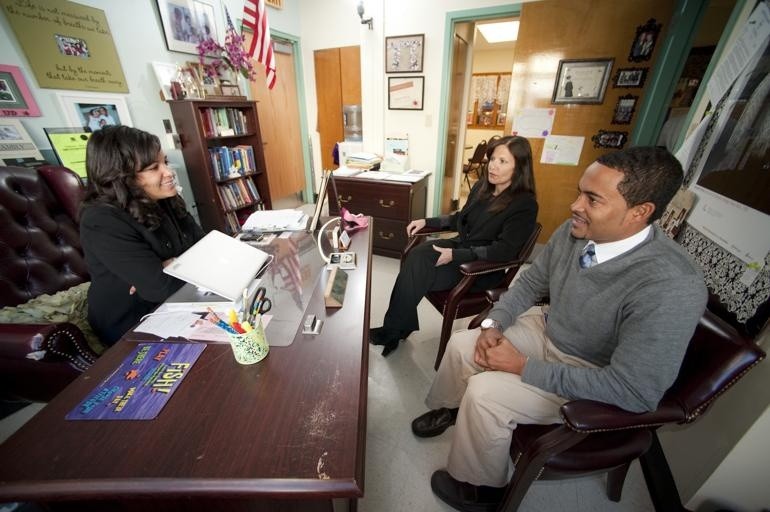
[356,0,373,30]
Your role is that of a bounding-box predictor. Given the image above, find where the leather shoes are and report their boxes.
[429,469,508,512]
[410,404,459,438]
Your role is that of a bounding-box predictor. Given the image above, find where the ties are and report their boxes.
[578,244,596,268]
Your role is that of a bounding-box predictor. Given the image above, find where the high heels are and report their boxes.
[368,325,400,358]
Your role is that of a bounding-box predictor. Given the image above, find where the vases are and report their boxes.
[230,70,248,101]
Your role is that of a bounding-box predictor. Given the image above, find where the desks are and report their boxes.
[0,215,373,511]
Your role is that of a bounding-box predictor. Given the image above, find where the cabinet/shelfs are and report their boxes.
[165,100,272,238]
[321,169,432,259]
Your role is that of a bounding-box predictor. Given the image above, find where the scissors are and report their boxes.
[247,287,272,326]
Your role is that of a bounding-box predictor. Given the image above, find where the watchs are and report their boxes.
[479,318,503,332]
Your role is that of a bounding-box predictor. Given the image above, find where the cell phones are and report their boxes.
[240,233,263,242]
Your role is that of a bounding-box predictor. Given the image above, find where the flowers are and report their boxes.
[196,26,257,83]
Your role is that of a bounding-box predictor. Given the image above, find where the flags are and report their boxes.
[242,1,276,90]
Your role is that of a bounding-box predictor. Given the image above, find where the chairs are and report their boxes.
[0,165,104,404]
[468,287,766,512]
[463,139,487,192]
[399,222,543,372]
[468,135,501,177]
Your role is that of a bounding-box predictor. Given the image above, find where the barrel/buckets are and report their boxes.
[342,103,362,143]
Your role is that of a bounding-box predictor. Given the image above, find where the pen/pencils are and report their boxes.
[206,306,238,336]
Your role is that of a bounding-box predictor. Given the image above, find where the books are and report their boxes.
[331,149,432,184]
[196,104,267,236]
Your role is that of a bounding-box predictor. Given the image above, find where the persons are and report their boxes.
[62,39,87,56]
[170,5,215,47]
[366,135,539,358]
[631,32,654,57]
[77,124,207,349]
[0,81,12,100]
[410,143,711,511]
[86,105,118,130]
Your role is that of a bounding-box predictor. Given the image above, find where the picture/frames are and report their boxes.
[612,67,650,89]
[0,64,42,117]
[186,61,223,96]
[220,85,241,96]
[385,34,424,73]
[56,91,134,133]
[628,17,663,63]
[151,61,196,101]
[591,129,629,149]
[610,93,640,126]
[387,76,425,110]
[550,57,615,105]
